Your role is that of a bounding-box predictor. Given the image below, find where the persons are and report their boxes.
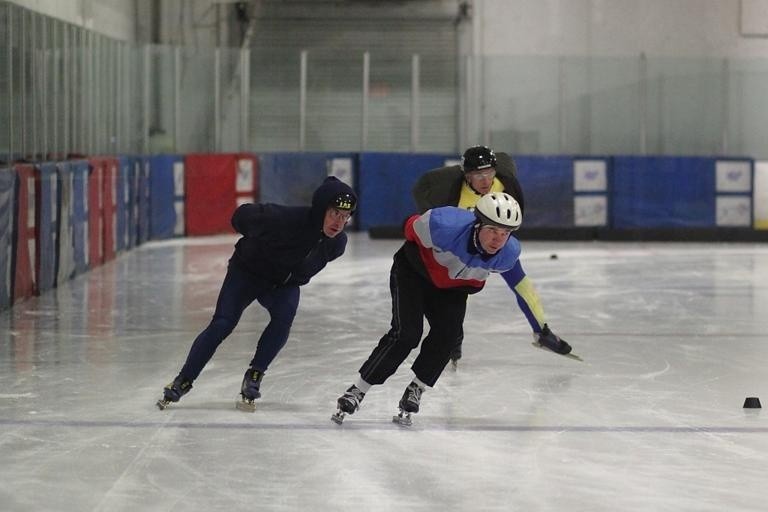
[162,176,357,402]
[337,191,524,415]
[416,146,573,359]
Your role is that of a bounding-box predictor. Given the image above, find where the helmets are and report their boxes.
[474,192,522,230]
[460,144,497,175]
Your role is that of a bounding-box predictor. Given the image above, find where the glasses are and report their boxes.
[474,171,496,179]
[331,209,352,223]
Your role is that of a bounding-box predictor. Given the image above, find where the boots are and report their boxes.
[338,384,365,415]
[451,334,462,360]
[164,373,193,402]
[534,324,572,354]
[242,369,265,399]
[399,382,422,413]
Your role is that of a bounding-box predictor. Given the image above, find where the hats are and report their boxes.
[329,192,356,211]
